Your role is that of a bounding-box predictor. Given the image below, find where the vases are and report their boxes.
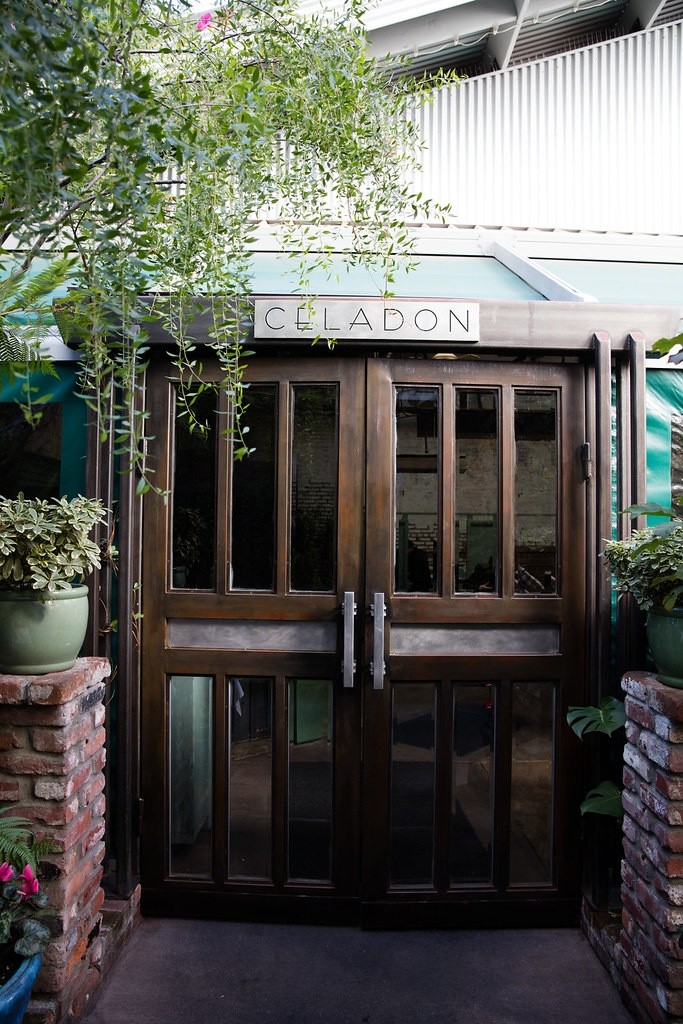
[0,953,42,1024]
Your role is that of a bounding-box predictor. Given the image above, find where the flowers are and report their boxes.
[0,863,50,973]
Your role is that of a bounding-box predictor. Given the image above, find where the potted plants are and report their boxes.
[597,524,683,688]
[0,491,119,675]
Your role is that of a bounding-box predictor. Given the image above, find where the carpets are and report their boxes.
[289,762,490,885]
[395,703,532,756]
